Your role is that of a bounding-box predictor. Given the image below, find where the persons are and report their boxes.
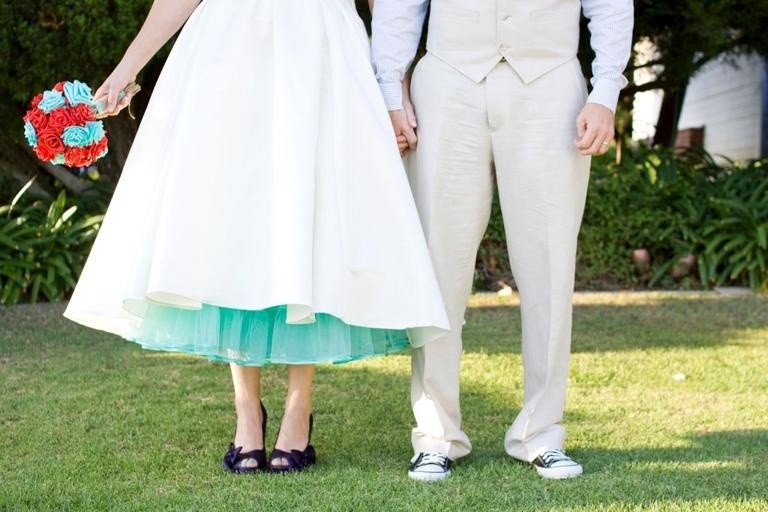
[370,1,636,481]
[62,0,455,471]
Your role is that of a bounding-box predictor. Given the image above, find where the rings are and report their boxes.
[602,142,610,147]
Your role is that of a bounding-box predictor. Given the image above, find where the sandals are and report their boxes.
[223,400,267,472]
[269,413,313,472]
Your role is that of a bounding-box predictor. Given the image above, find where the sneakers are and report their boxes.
[407,452,453,481]
[532,448,583,479]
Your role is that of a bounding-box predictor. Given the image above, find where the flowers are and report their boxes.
[21,79,140,171]
